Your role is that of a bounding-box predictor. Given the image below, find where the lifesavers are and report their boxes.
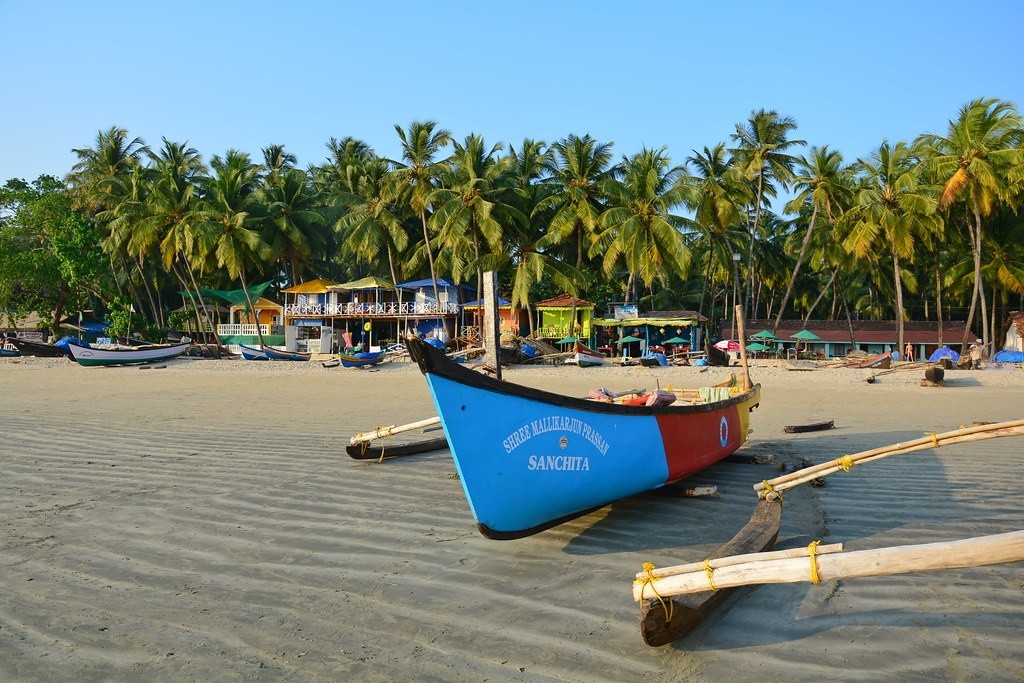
[364,322,371,331]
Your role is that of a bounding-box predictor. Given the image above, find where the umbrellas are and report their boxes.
[663,337,690,358]
[614,335,644,360]
[792,330,820,351]
[713,339,739,353]
[745,330,778,359]
[554,336,584,353]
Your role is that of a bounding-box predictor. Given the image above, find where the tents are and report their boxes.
[994,349,1024,363]
[280,275,475,315]
[929,346,961,361]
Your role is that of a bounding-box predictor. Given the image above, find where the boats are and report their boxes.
[640,357,662,366]
[8,337,72,357]
[405,304,761,543]
[340,348,387,367]
[846,352,893,368]
[262,343,312,361]
[239,342,270,361]
[68,336,192,366]
[573,338,606,368]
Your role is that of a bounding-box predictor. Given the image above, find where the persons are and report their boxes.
[357,340,362,352]
[905,342,914,361]
[969,338,993,370]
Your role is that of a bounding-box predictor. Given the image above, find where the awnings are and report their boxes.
[590,317,699,333]
